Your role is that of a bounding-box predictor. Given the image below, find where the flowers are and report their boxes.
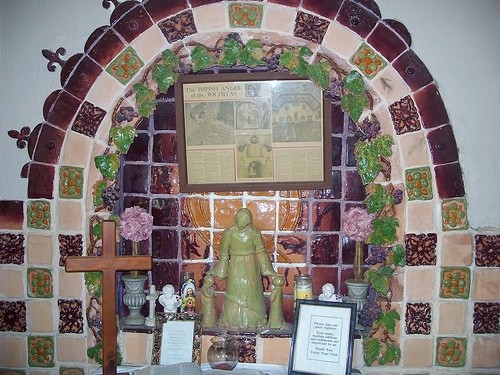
[341,208,377,279]
[112,207,153,278]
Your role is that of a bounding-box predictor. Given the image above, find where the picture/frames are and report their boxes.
[175,73,334,193]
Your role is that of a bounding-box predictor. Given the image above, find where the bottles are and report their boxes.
[293,274,312,313]
[207,336,239,370]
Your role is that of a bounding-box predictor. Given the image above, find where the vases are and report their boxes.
[342,277,371,331]
[120,275,148,326]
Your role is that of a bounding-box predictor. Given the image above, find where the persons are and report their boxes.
[201,275,219,326]
[159,284,182,312]
[180,288,201,316]
[318,283,342,303]
[267,277,286,328]
[189,103,209,121]
[206,208,284,330]
